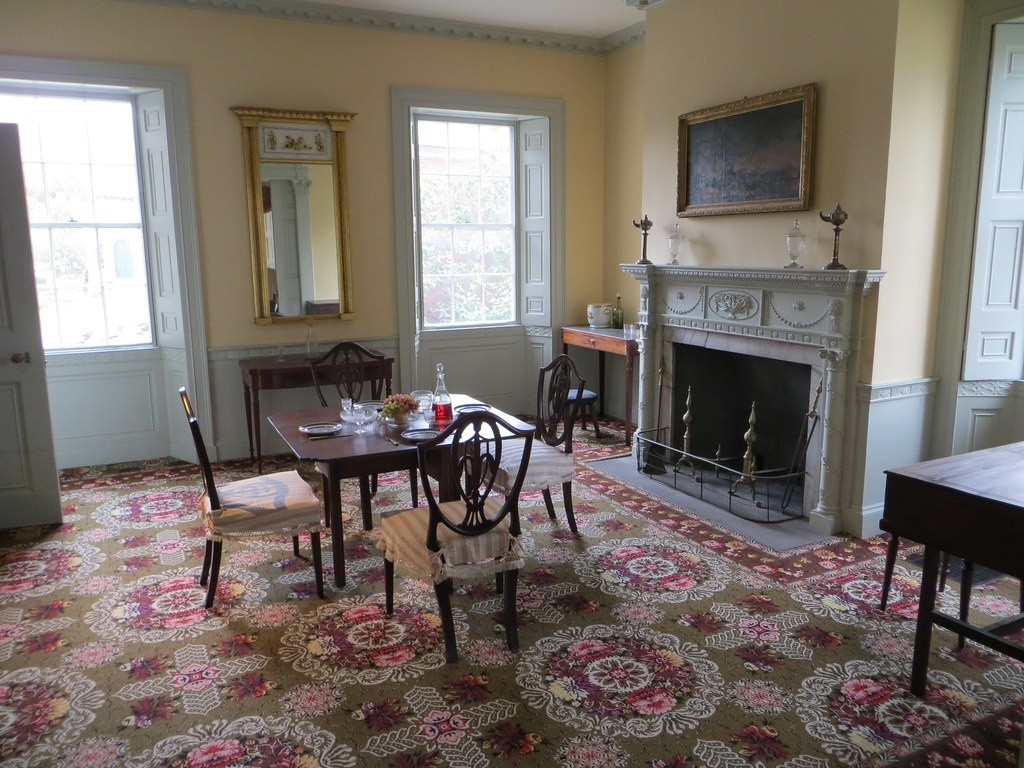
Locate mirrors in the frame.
[228,106,359,326]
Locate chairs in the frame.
[465,353,585,533]
[381,411,536,665]
[178,386,325,609]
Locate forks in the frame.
[383,433,398,445]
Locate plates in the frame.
[454,404,491,414]
[339,410,378,423]
[354,400,384,410]
[298,422,342,435]
[401,428,443,441]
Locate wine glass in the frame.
[352,410,366,434]
[667,223,685,265]
[784,219,806,269]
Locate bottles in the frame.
[305,323,320,360]
[613,296,623,329]
[432,363,453,425]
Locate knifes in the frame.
[309,434,354,440]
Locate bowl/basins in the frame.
[411,390,433,411]
[587,303,613,328]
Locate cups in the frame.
[341,398,352,411]
[423,409,436,423]
[624,323,638,340]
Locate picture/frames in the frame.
[676,82,816,218]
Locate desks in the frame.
[239,349,395,475]
[308,341,418,530]
[560,326,637,446]
[876,440,1024,701]
[266,393,534,588]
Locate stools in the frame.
[565,389,602,438]
[879,519,1024,648]
